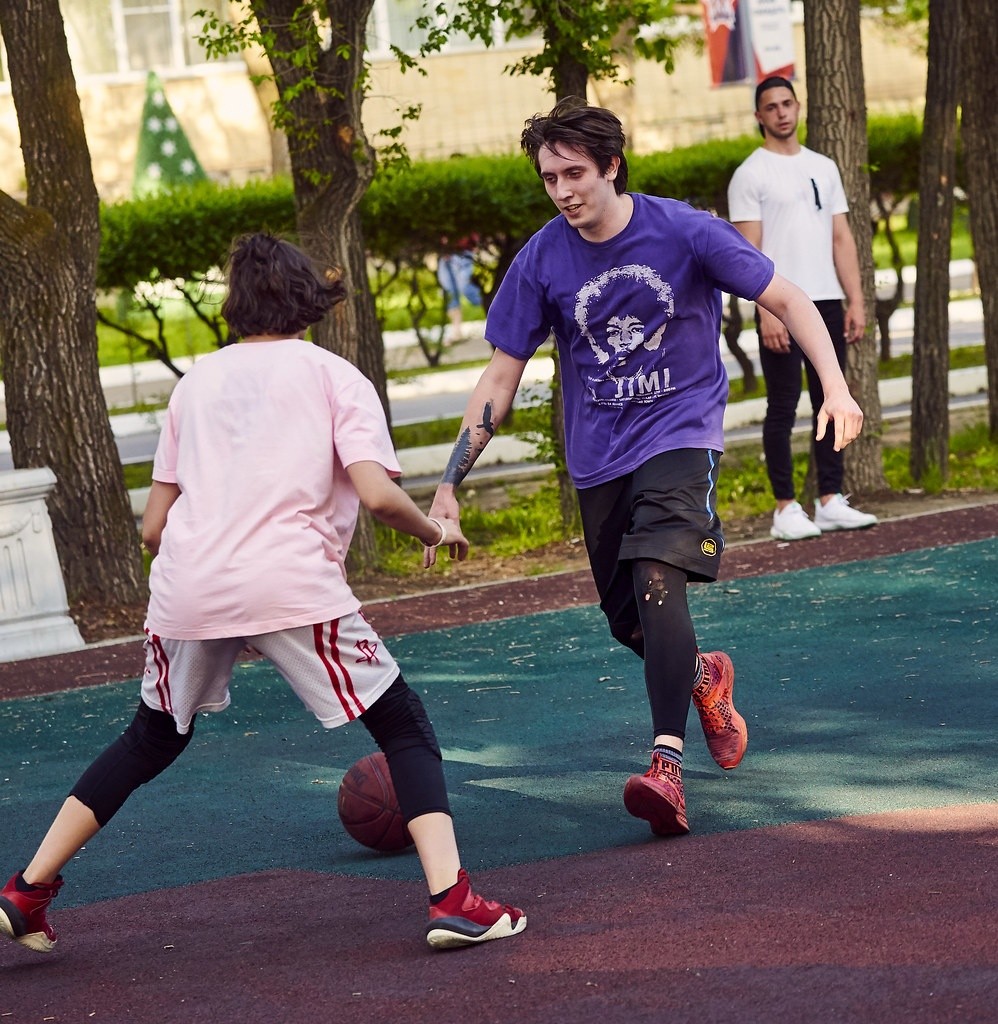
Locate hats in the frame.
[756,76,795,140]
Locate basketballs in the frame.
[336,751,419,851]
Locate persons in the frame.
[0,234,527,951]
[433,227,482,346]
[429,104,864,836]
[727,77,878,540]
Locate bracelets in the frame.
[422,517,446,549]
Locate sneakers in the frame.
[813,492,878,532]
[426,867,528,948]
[693,650,748,770]
[623,752,690,836]
[770,499,822,541]
[0,869,65,952]
